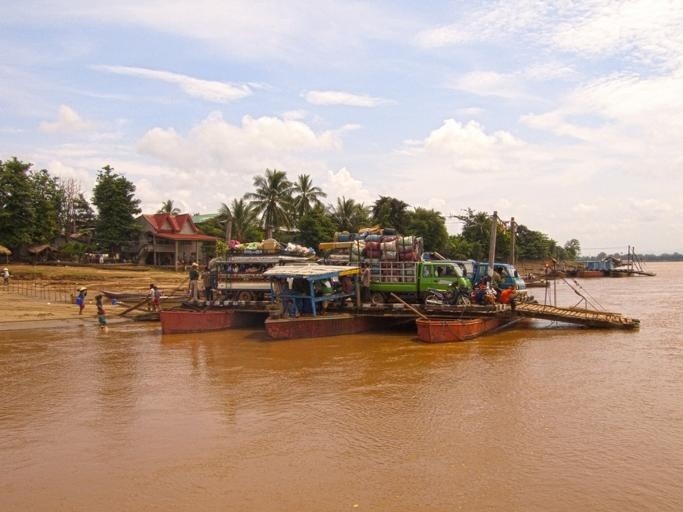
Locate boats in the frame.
[416,315,524,342]
[102,290,187,303]
[526,269,604,287]
[264,314,413,339]
[160,306,259,334]
[613,271,634,277]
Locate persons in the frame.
[153,286,162,312]
[285,277,354,312]
[75,286,87,315]
[200,267,214,301]
[94,293,105,315]
[185,262,200,300]
[0,267,9,286]
[469,268,520,303]
[283,299,298,319]
[147,283,157,312]
[434,267,443,276]
[218,263,273,275]
[79,250,120,264]
[359,261,370,302]
[445,267,453,274]
